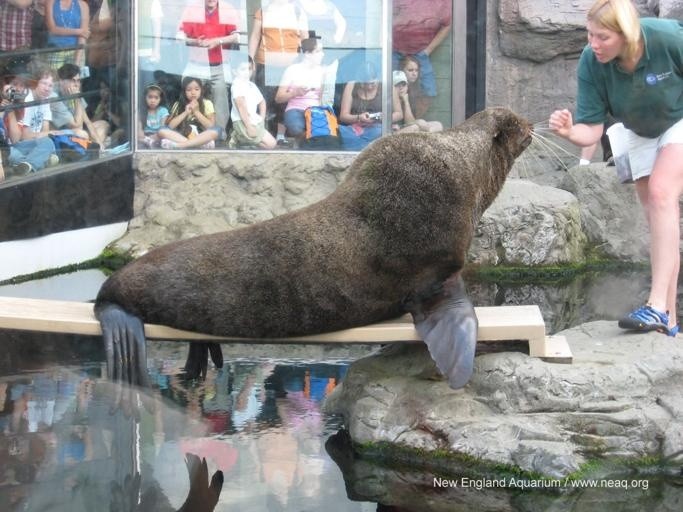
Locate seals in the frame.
[93,105,586,424]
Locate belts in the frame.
[190,61,221,66]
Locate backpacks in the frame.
[48,132,91,161]
[294,105,342,152]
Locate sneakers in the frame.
[200,140,215,149]
[220,129,227,141]
[228,136,236,149]
[145,137,154,148]
[88,143,101,162]
[160,138,173,150]
[12,153,59,176]
[617,306,668,331]
[276,133,285,145]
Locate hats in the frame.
[392,70,407,85]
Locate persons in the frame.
[578,111,618,166]
[548,1,683,336]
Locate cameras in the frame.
[368,111,382,120]
[7,88,25,104]
